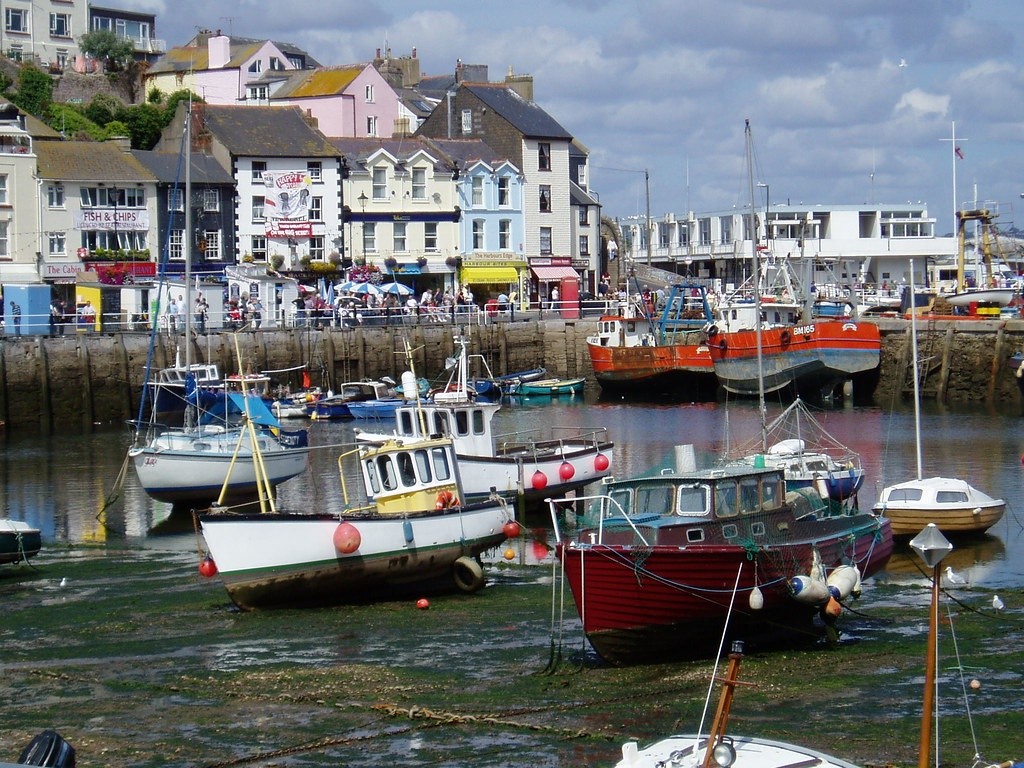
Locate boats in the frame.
[0,518,42,566]
[353,270,614,512]
[873,252,1008,543]
[190,324,515,611]
[543,468,895,670]
[613,524,1024,768]
[223,287,586,418]
[586,121,1024,398]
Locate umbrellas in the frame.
[350,281,382,294]
[334,281,358,292]
[378,281,414,302]
[300,277,334,303]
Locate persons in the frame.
[50,301,95,337]
[224,291,267,328]
[811,282,819,293]
[882,281,904,295]
[292,294,418,327]
[951,277,957,289]
[607,237,618,263]
[993,277,997,287]
[599,272,627,300]
[692,287,716,301]
[10,302,20,337]
[419,286,473,322]
[164,292,209,333]
[551,286,560,308]
[642,285,665,303]
[498,292,516,310]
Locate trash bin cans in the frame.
[488,299,498,317]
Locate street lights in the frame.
[356,191,369,266]
[110,186,121,264]
[756,182,769,293]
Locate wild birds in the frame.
[899,56,909,68]
[993,595,1006,614]
[944,567,969,587]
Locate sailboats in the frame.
[586,118,866,528]
[124,52,309,504]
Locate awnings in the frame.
[461,267,580,284]
